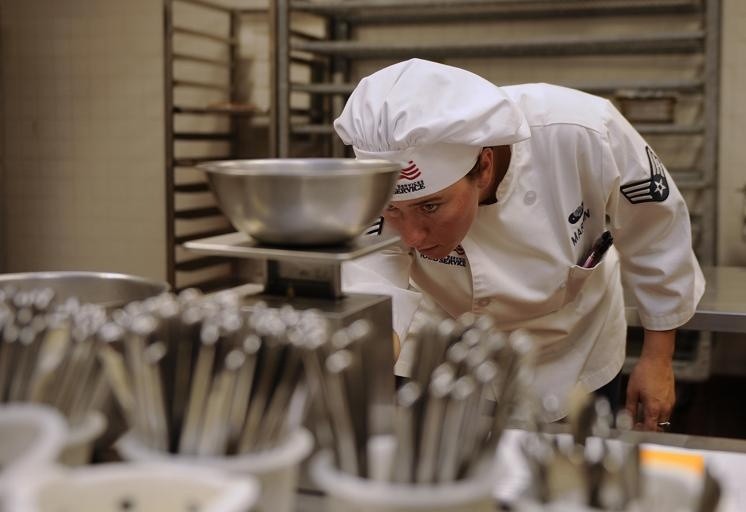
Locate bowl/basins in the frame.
[0,268,170,316]
[196,156,402,247]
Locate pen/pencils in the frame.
[582,231,613,271]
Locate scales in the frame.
[182,157,405,420]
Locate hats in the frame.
[332,56,532,203]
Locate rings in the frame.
[656,420,671,429]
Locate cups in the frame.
[107,421,317,509]
[25,467,256,512]
[307,430,517,510]
[2,402,55,511]
[500,465,723,512]
[52,411,105,463]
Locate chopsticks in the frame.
[1,282,533,483]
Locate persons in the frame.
[340,56,705,434]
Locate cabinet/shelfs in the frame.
[279,4,721,271]
